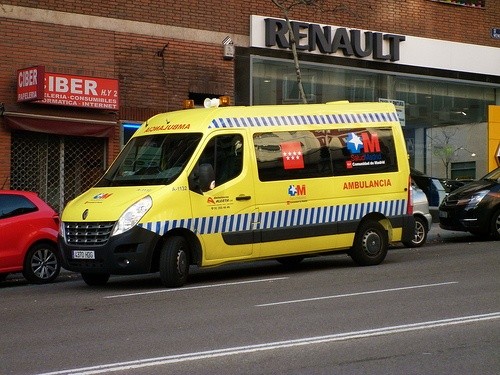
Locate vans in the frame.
[56,93,414,287]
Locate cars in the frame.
[0,188,63,284]
[411,180,433,247]
[437,166,500,241]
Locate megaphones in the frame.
[204,98,219,108]
